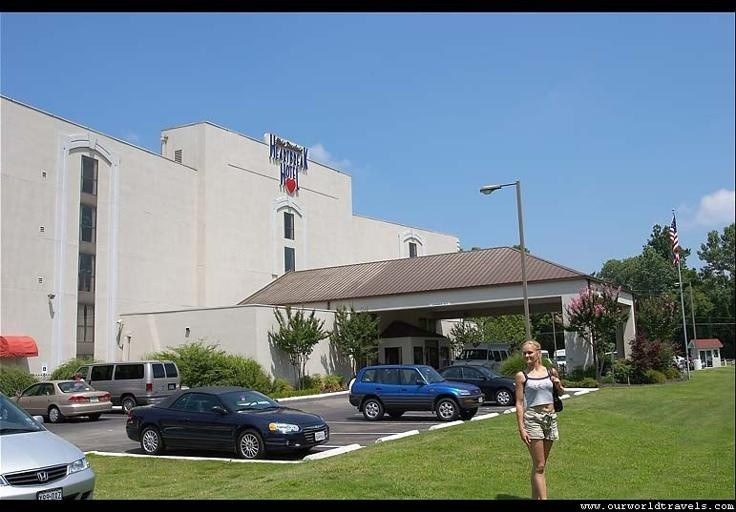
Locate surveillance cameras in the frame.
[116,320,124,326]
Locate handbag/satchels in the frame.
[553,383,563,411]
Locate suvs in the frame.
[349,364,485,422]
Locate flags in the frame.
[668,215,679,267]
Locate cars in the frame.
[9,379,112,420]
[0,392,95,502]
[126,386,329,459]
[437,365,517,406]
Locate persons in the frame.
[516,341,564,500]
[72,373,86,389]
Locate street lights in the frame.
[675,281,696,339]
[479,180,531,340]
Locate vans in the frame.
[540,349,567,367]
[452,341,511,369]
[73,359,179,413]
